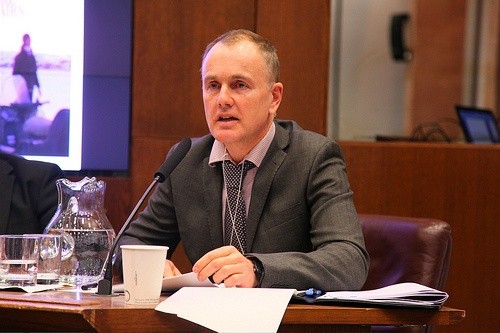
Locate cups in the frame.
[23,234,63,284]
[120,244,170,304]
[0,235,39,287]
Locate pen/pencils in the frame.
[81,282,98,290]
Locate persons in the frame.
[0,150,67,268]
[112,29,370,292]
[12,34,43,104]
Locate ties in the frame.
[222,159,255,254]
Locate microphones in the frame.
[96,137,193,294]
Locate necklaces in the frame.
[222,147,244,257]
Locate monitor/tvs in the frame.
[454,104,500,144]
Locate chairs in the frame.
[358,213,453,294]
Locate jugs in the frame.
[40,176,117,288]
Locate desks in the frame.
[336,141,500,333]
[0,286,465,333]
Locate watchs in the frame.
[250,258,266,283]
